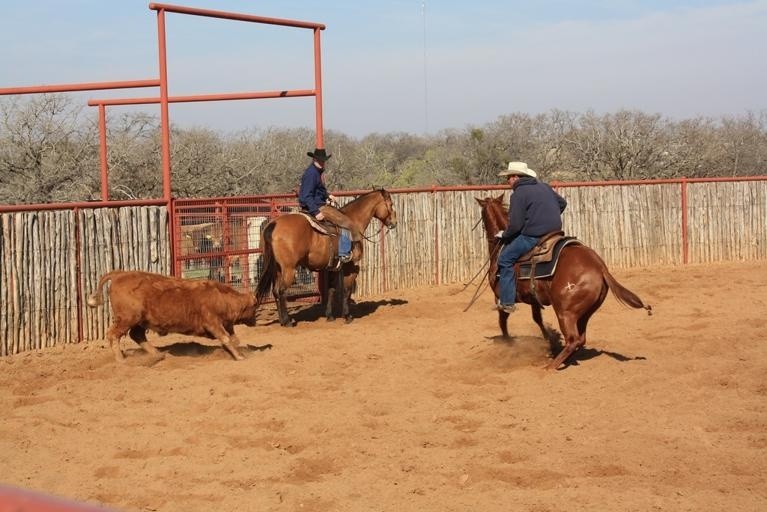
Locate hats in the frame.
[307,148,332,162]
[496,161,538,178]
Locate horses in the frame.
[253,185,398,327]
[474,191,644,372]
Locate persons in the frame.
[492,161,567,314]
[298,149,352,264]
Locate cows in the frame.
[86,269,257,361]
[181,217,312,284]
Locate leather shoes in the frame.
[491,302,515,312]
